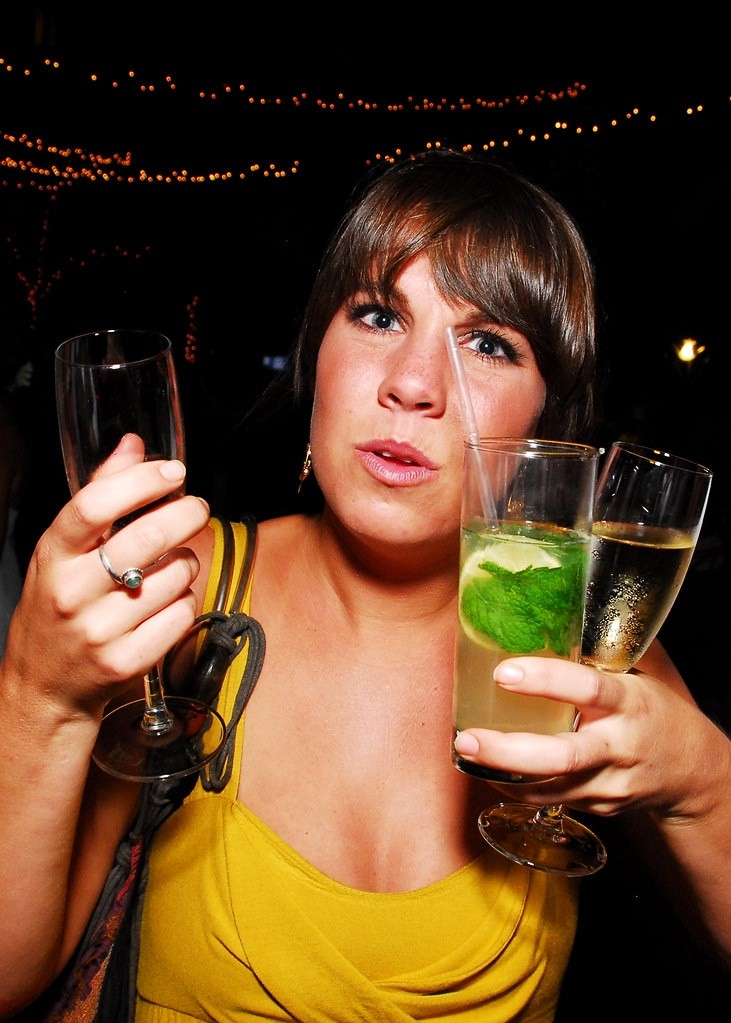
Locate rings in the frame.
[98,545,143,588]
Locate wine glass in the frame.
[477,442,716,879]
[54,328,226,783]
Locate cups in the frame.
[449,438,599,785]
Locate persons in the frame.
[0,148,731,1023]
